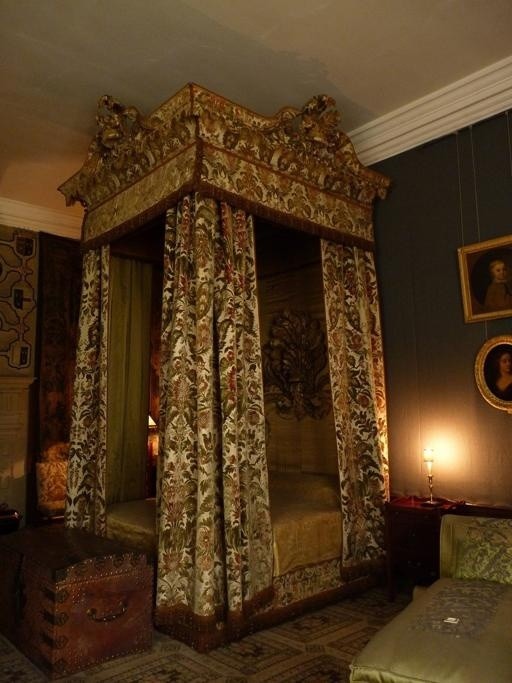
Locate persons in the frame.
[493,351,512,402]
[484,260,512,312]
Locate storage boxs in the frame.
[0,524,154,680]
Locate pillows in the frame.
[267,470,341,507]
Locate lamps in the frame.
[420,475,443,507]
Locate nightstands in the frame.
[383,496,464,602]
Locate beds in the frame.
[57,82,391,653]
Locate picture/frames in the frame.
[474,335,512,415]
[456,234,512,325]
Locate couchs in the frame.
[348,515,512,683]
